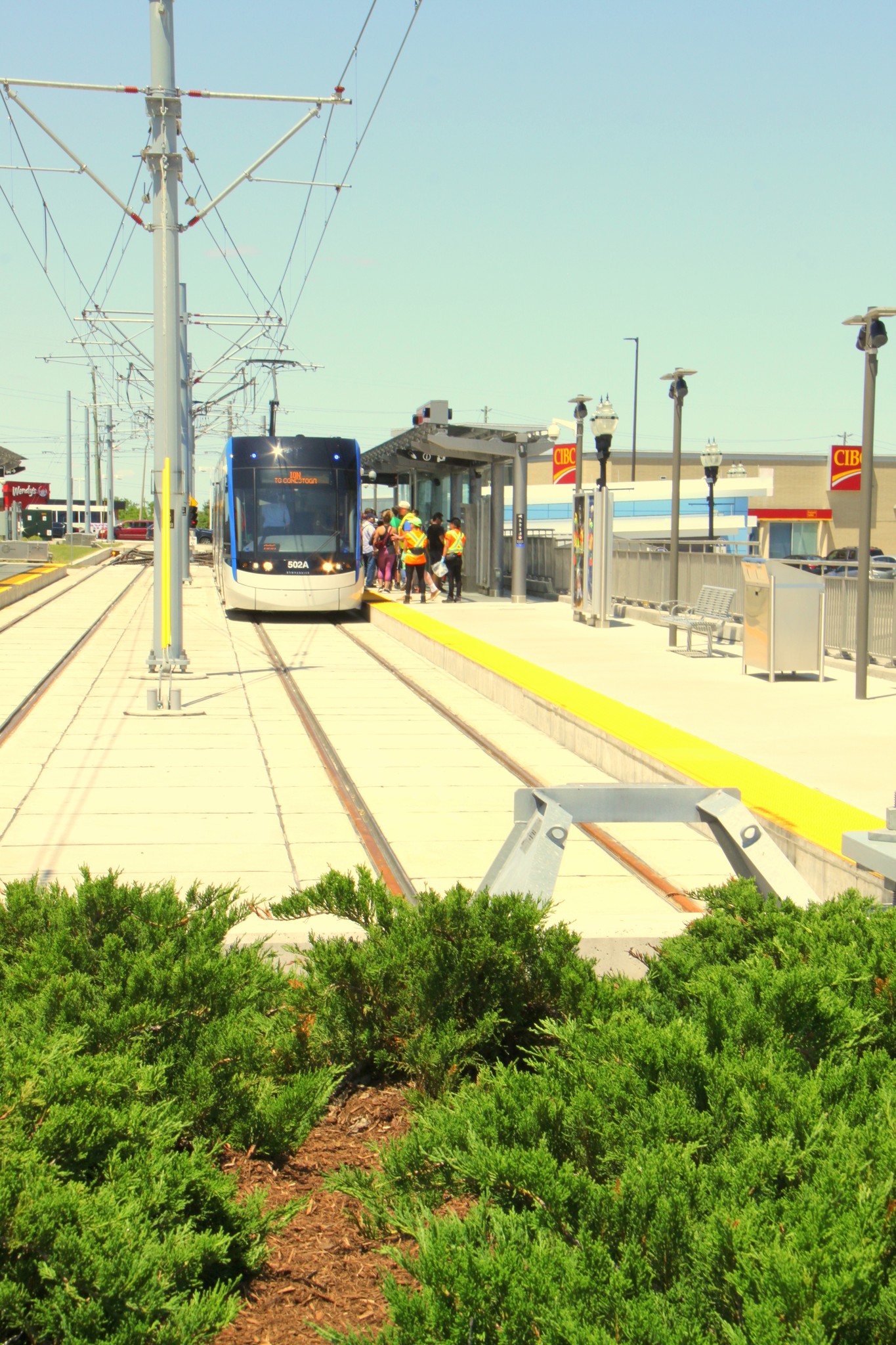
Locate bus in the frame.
[210,434,365,615]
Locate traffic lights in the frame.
[187,506,198,529]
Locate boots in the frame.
[421,595,426,603]
[403,596,410,604]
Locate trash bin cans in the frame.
[742,557,826,671]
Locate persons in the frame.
[372,510,401,594]
[361,508,425,593]
[361,513,377,588]
[262,495,290,536]
[426,512,447,593]
[439,517,466,603]
[390,500,442,602]
[402,518,430,604]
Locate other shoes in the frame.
[394,582,401,589]
[366,585,377,588]
[401,583,406,591]
[378,586,390,593]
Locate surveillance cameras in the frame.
[368,471,377,482]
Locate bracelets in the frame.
[397,538,399,540]
[373,550,376,552]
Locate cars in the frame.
[52,522,80,538]
[780,555,825,576]
[822,555,896,580]
[146,521,213,545]
[97,520,154,541]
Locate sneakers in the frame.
[454,596,461,603]
[442,596,454,603]
[396,595,412,600]
[428,589,441,601]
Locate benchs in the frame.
[660,584,735,658]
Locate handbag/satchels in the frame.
[408,547,424,555]
[431,561,448,577]
[374,527,392,550]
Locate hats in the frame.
[365,513,378,520]
[408,518,422,527]
[446,517,461,528]
[398,501,410,509]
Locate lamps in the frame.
[518,442,527,457]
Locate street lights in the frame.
[698,436,723,553]
[567,394,592,608]
[841,306,896,703]
[622,336,639,482]
[659,367,699,647]
[590,394,620,492]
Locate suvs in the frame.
[825,546,885,572]
